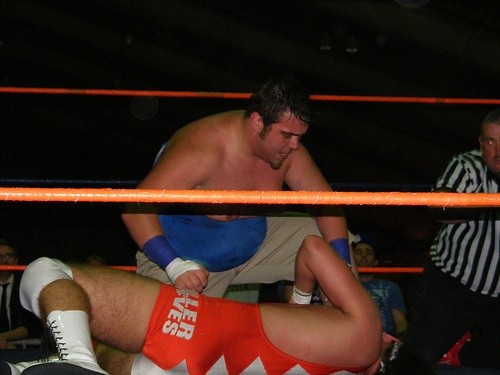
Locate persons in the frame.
[399,107,500,368]
[0,234,436,375]
[354,240,407,332]
[0,241,43,349]
[120,74,359,305]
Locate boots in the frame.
[0,353,59,375]
[20,310,110,375]
